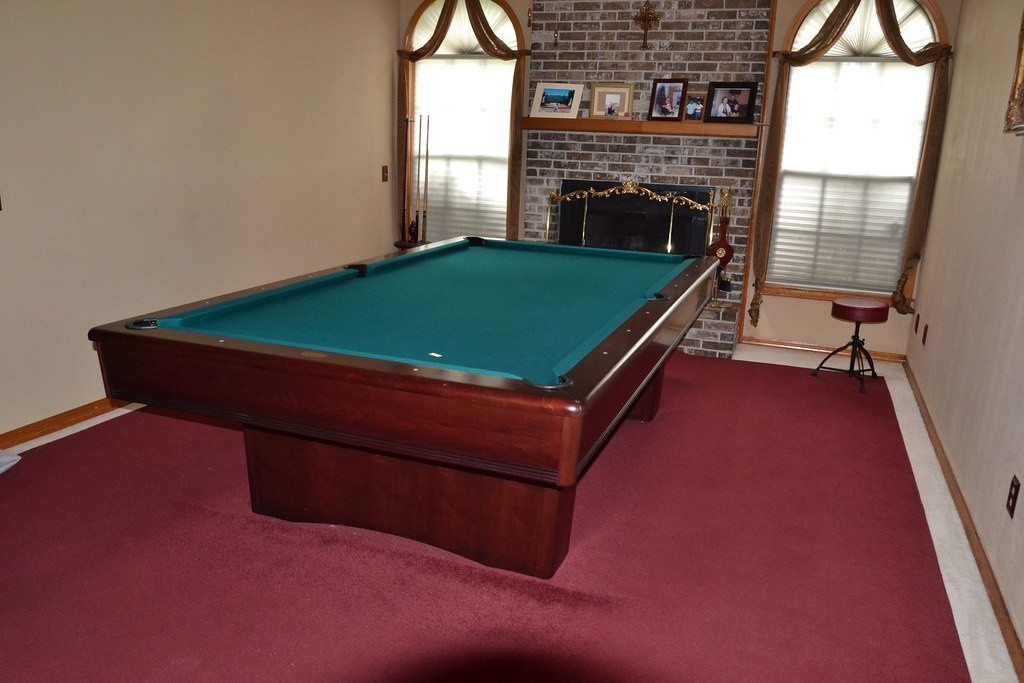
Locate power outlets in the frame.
[1005,474,1020,520]
[922,323,928,346]
[915,313,920,333]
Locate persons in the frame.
[553,102,559,112]
[686,99,703,120]
[717,96,740,117]
[607,105,615,116]
[660,96,675,117]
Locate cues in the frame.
[414,115,422,245]
[401,113,408,243]
[422,114,430,243]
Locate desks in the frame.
[88,236,721,581]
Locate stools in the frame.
[810,298,890,396]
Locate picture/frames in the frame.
[647,78,689,121]
[683,92,707,123]
[529,82,585,119]
[589,83,635,121]
[702,81,759,123]
